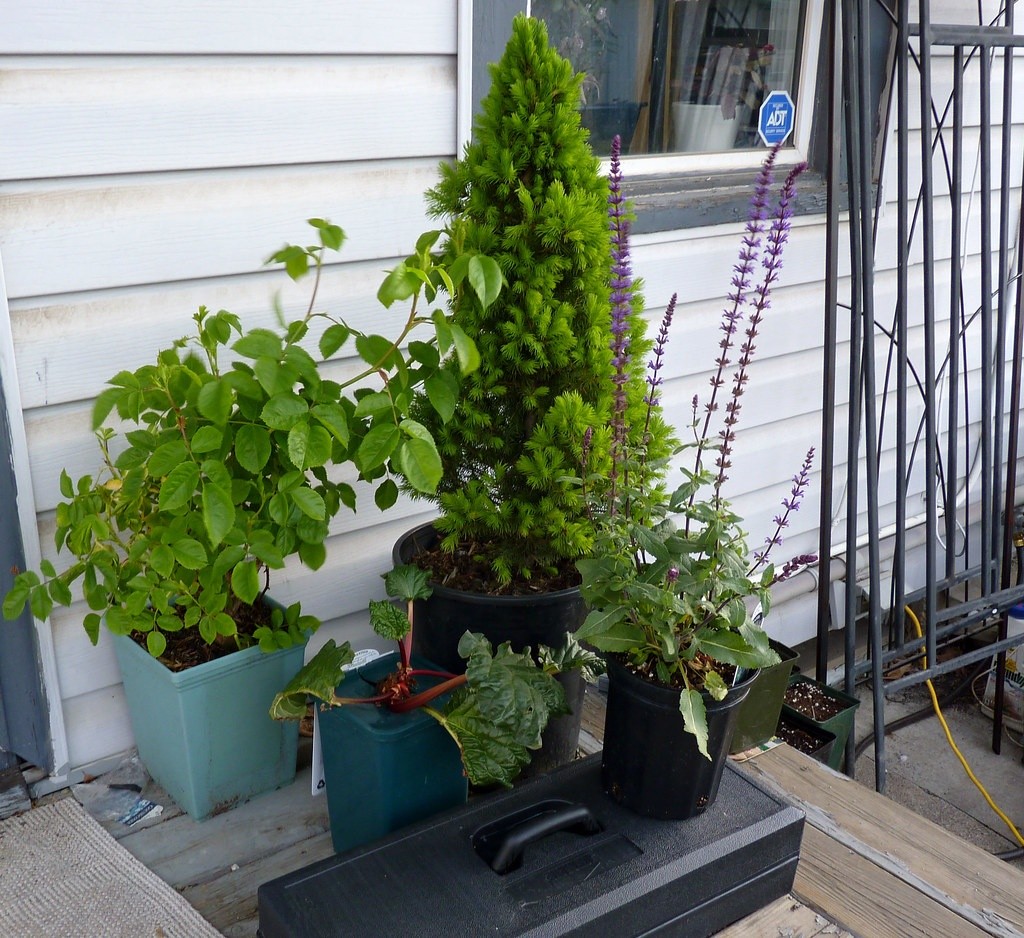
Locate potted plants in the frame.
[0,214,509,817]
[670,42,775,150]
[385,14,683,785]
[277,552,565,847]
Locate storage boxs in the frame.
[251,725,808,938]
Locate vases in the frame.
[728,635,803,748]
[785,674,861,775]
[601,626,761,821]
[778,712,838,768]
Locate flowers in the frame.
[572,127,816,772]
